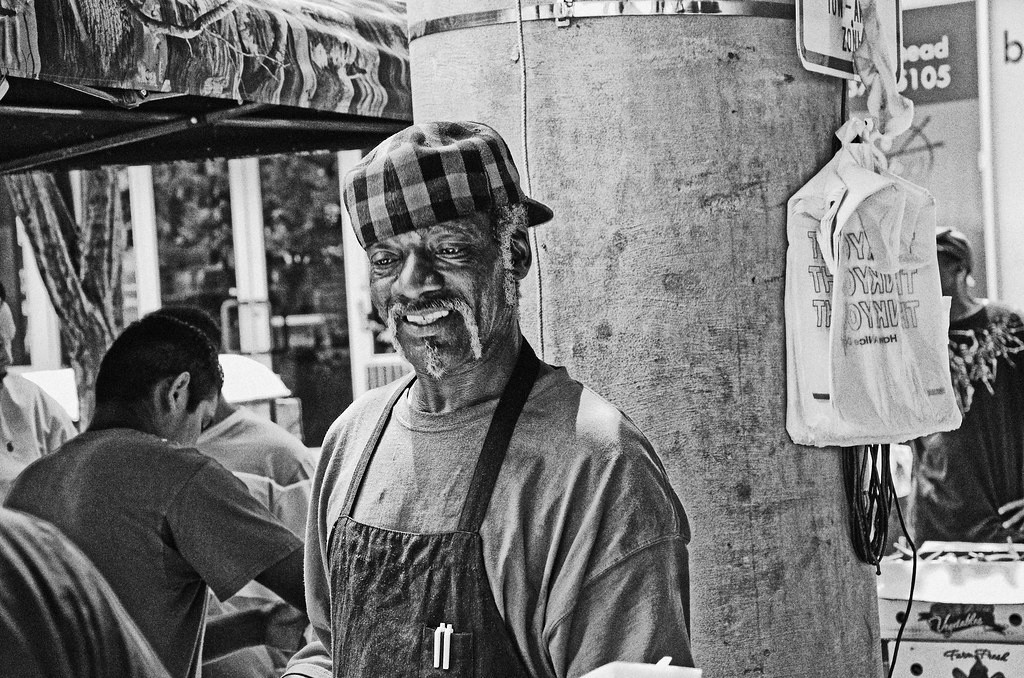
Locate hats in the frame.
[342,121,554,252]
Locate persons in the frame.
[0,121,690,678]
[906,225,1024,549]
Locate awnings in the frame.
[0,0,413,121]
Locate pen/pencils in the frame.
[433,623,454,669]
